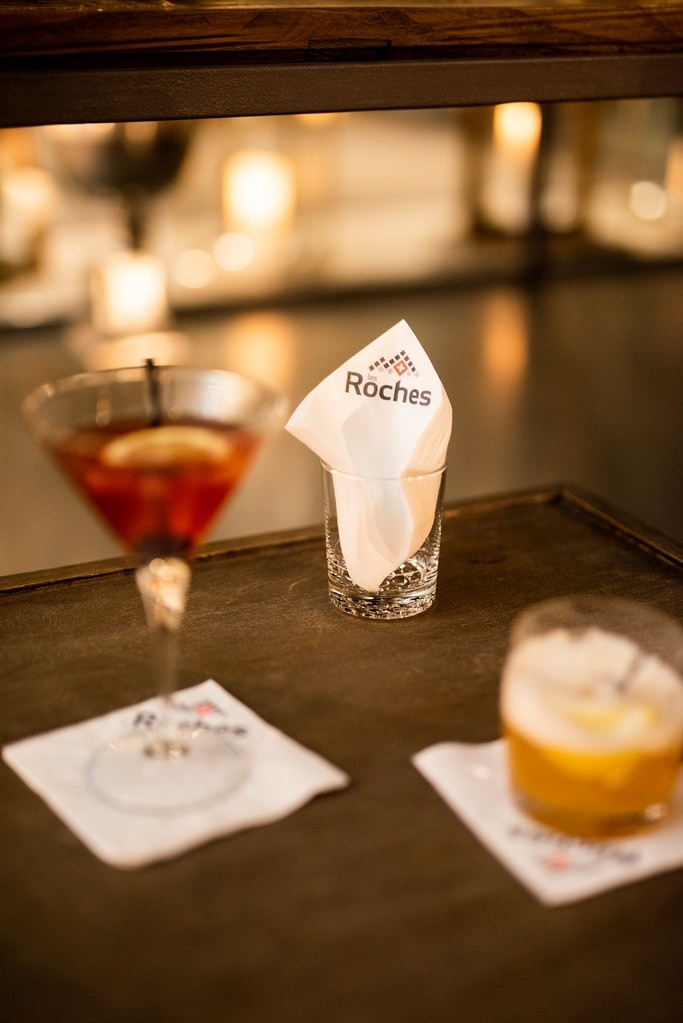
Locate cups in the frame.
[502,592,682,841]
[317,456,448,621]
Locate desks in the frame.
[0,479,683,1023]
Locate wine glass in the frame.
[21,354,287,814]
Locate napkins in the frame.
[285,318,452,591]
[410,731,683,909]
[1,679,350,872]
